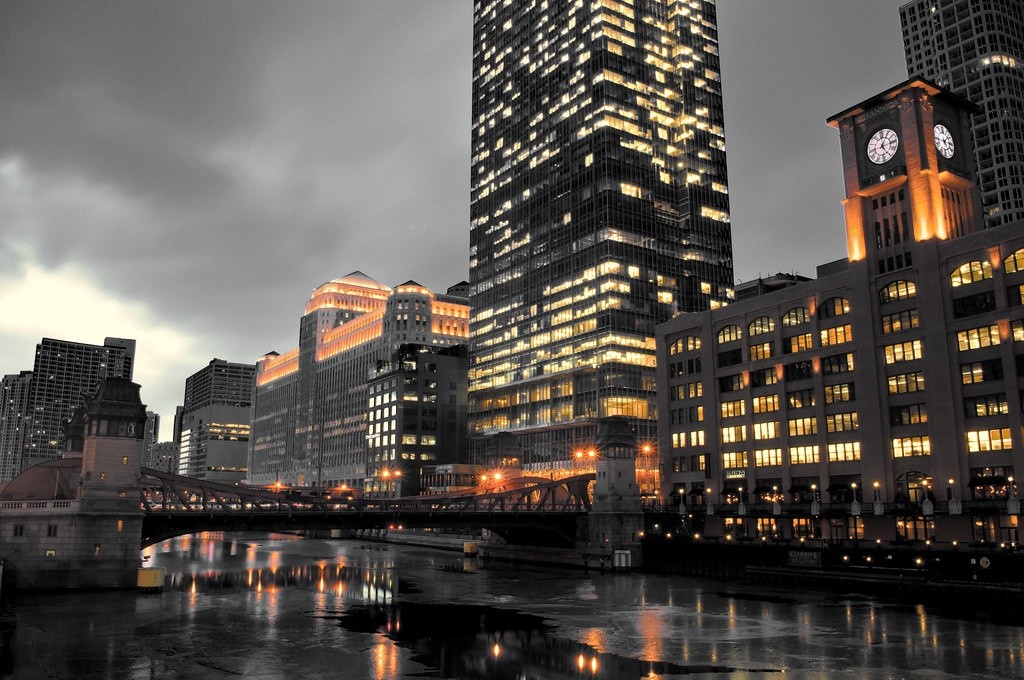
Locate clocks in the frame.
[934,124,955,159]
[867,129,899,165]
